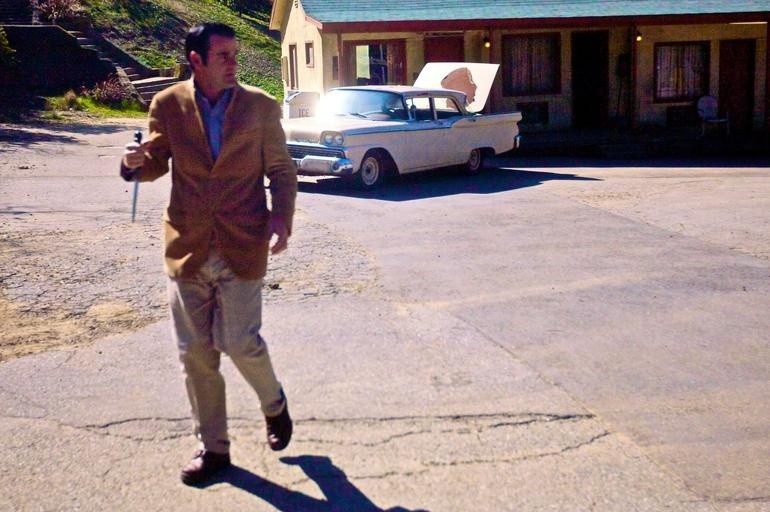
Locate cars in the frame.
[279,61,523,190]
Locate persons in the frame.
[119,22,299,485]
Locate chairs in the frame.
[696,95,730,137]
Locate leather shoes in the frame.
[182,450,231,483]
[265,408,293,451]
